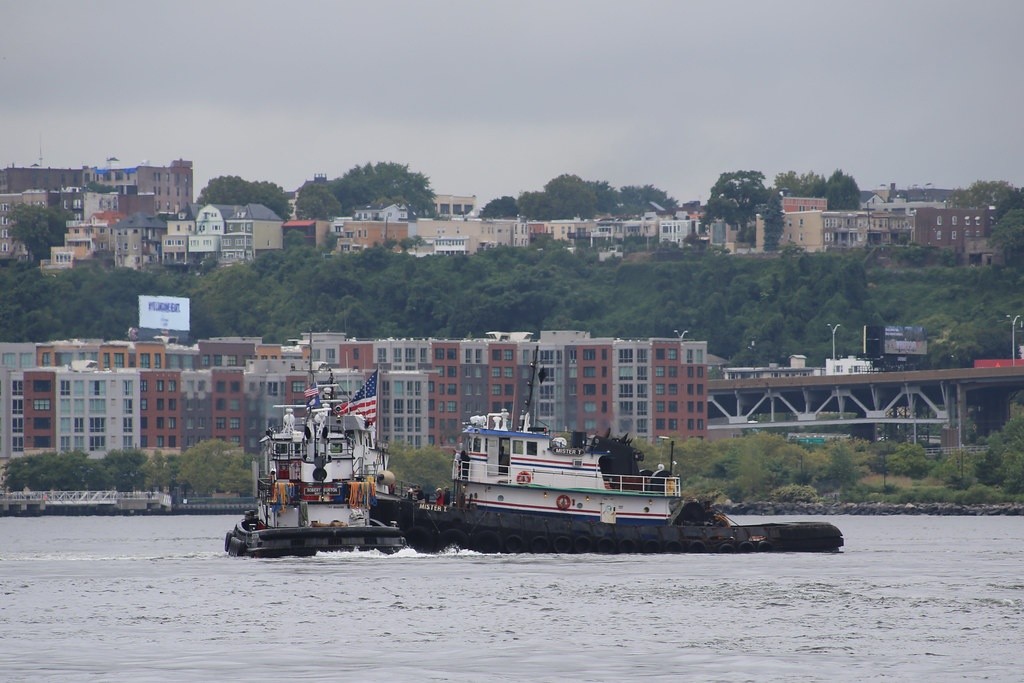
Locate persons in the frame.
[388,450,471,506]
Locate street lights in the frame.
[674,329,689,338]
[827,323,841,375]
[1006,314,1021,367]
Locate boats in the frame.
[372,346,845,555]
[226,331,405,558]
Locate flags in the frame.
[331,369,379,433]
[304,382,322,409]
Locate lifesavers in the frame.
[517,471,532,485]
[556,495,571,509]
